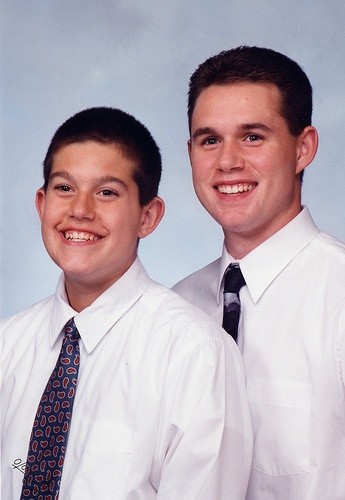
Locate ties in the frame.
[221,265,245,344]
[22,317,80,499]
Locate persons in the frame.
[169,44,345,500]
[0,107,253,500]
